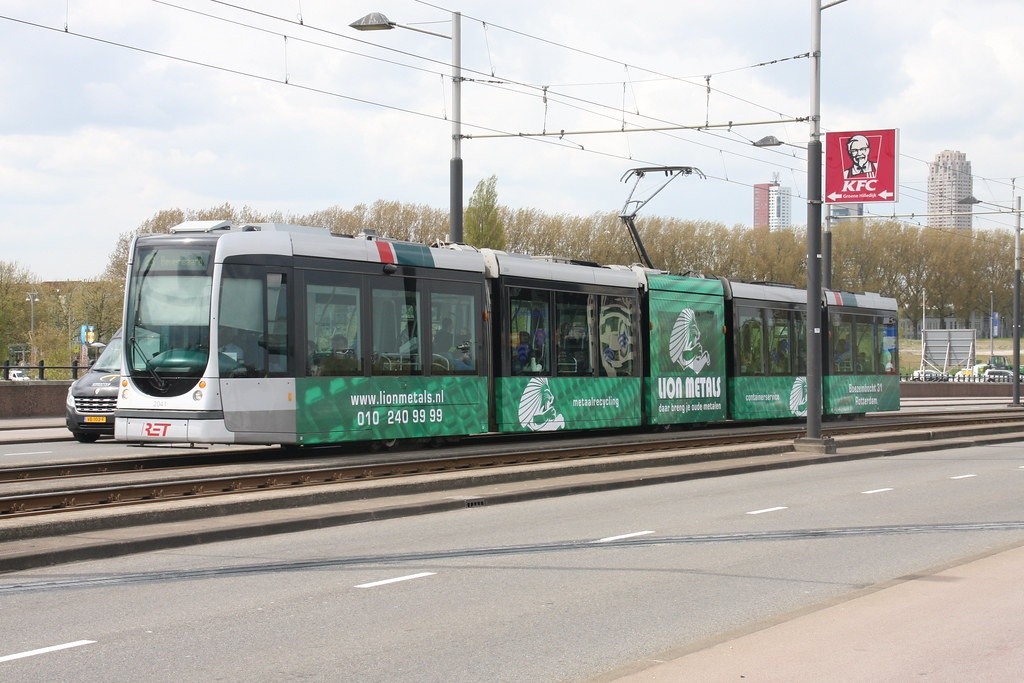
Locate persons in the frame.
[557,323,575,363]
[218,327,245,364]
[516,331,532,367]
[321,334,357,376]
[431,317,455,354]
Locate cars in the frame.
[910,356,1024,382]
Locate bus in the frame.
[114,221,901,452]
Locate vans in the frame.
[65,326,122,443]
[8,371,30,381]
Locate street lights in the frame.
[26,287,40,367]
[752,136,832,292]
[957,196,1024,407]
[348,12,464,245]
